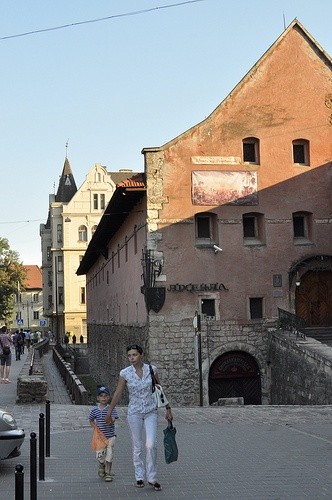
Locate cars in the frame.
[0,410,24,460]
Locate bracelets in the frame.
[166,406,171,410]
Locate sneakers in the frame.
[136,480,144,487]
[97,462,112,481]
[149,482,162,491]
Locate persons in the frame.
[63,331,72,344]
[0,326,14,384]
[80,335,84,344]
[72,334,77,345]
[12,326,42,360]
[89,387,119,482]
[105,344,173,492]
[48,329,55,342]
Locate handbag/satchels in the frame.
[92,425,109,451]
[0,338,10,355]
[162,420,178,464]
[149,365,169,408]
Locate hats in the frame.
[96,386,111,396]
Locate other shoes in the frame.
[1,378,11,384]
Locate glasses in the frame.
[126,345,138,349]
